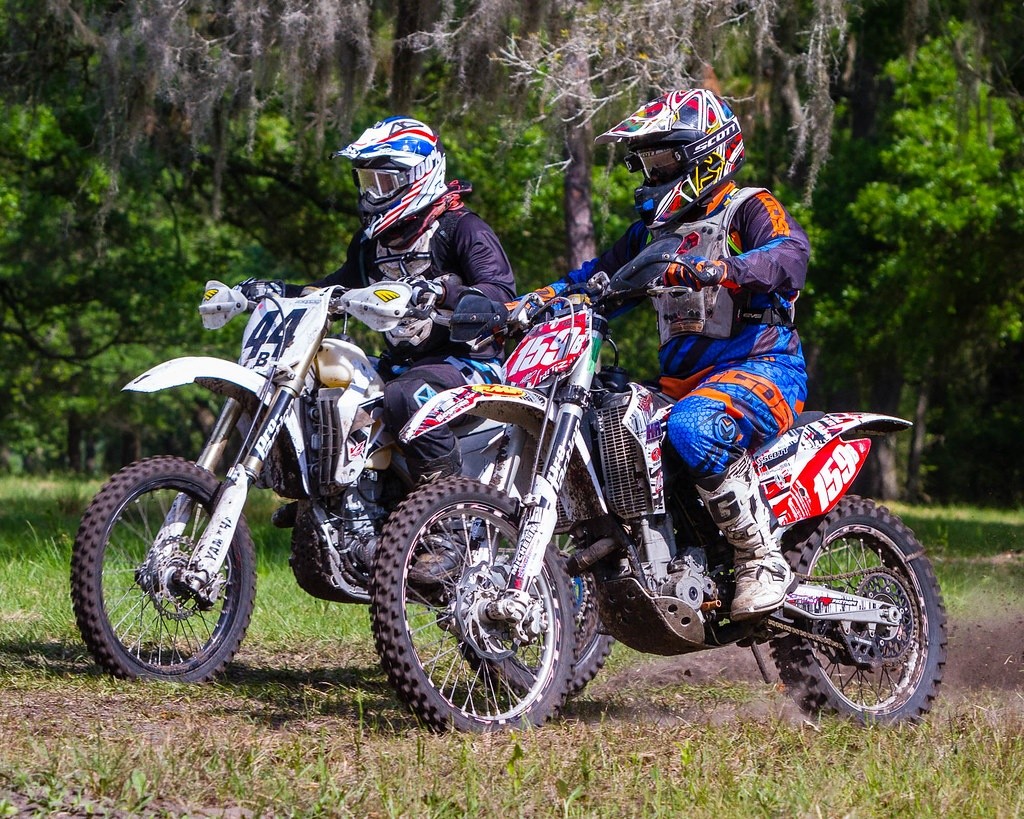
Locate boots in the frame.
[407,436,480,584]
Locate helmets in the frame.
[593,89,748,229]
[330,116,449,240]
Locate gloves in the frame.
[503,287,556,317]
[662,254,734,292]
[232,277,285,304]
[396,276,444,307]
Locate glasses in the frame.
[637,148,680,182]
[356,168,410,201]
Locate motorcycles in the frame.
[368,233,949,735]
[70,280,614,685]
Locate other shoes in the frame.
[695,449,799,621]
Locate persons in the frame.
[241,111,518,582]
[501,88,812,622]
